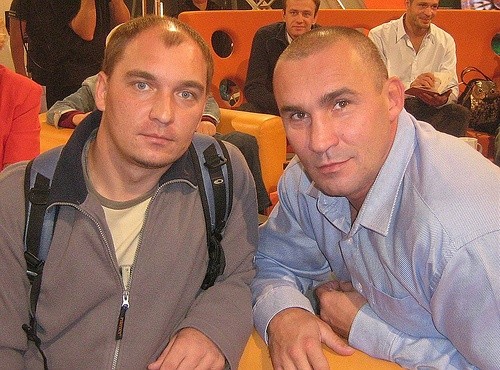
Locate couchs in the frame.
[38,105,287,199]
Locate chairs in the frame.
[238,326,404,370]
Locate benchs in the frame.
[179,8,499,158]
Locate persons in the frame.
[251,25,500,370]
[9,0,130,110]
[0,14,259,370]
[47,22,272,215]
[367,0,471,137]
[175,0,219,16]
[0,63,44,173]
[243,0,323,116]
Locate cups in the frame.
[459,137,482,154]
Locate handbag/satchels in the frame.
[458,64,500,136]
